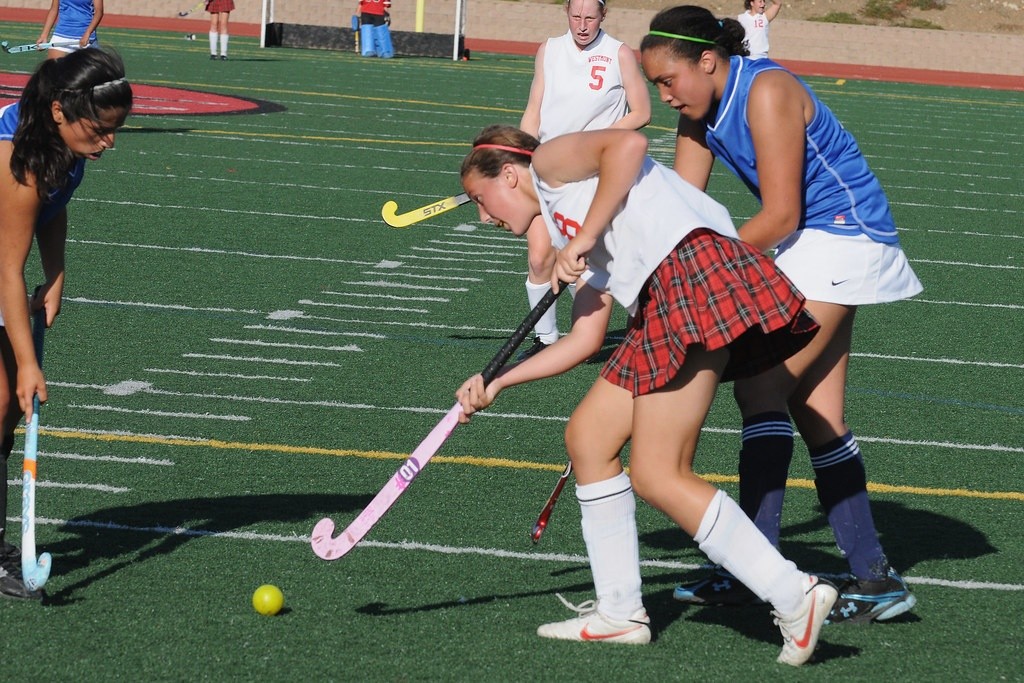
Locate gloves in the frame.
[383,12,392,26]
[351,14,361,32]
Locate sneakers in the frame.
[671,560,754,605]
[514,336,546,364]
[816,563,918,628]
[0,562,43,602]
[0,527,22,564]
[536,591,653,645]
[774,573,839,668]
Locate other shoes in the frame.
[209,54,217,61]
[382,51,395,60]
[220,54,228,60]
[364,51,377,59]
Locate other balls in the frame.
[254,584,284,616]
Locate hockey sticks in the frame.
[355,31,360,54]
[381,191,472,228]
[308,255,590,561]
[19,282,54,593]
[530,461,572,544]
[1,38,95,54]
[179,1,206,16]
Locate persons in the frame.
[640,5,923,620]
[36,0,104,57]
[455,126,839,668]
[0,48,134,601]
[204,0,236,61]
[737,0,782,60]
[351,0,394,58]
[516,0,651,358]
